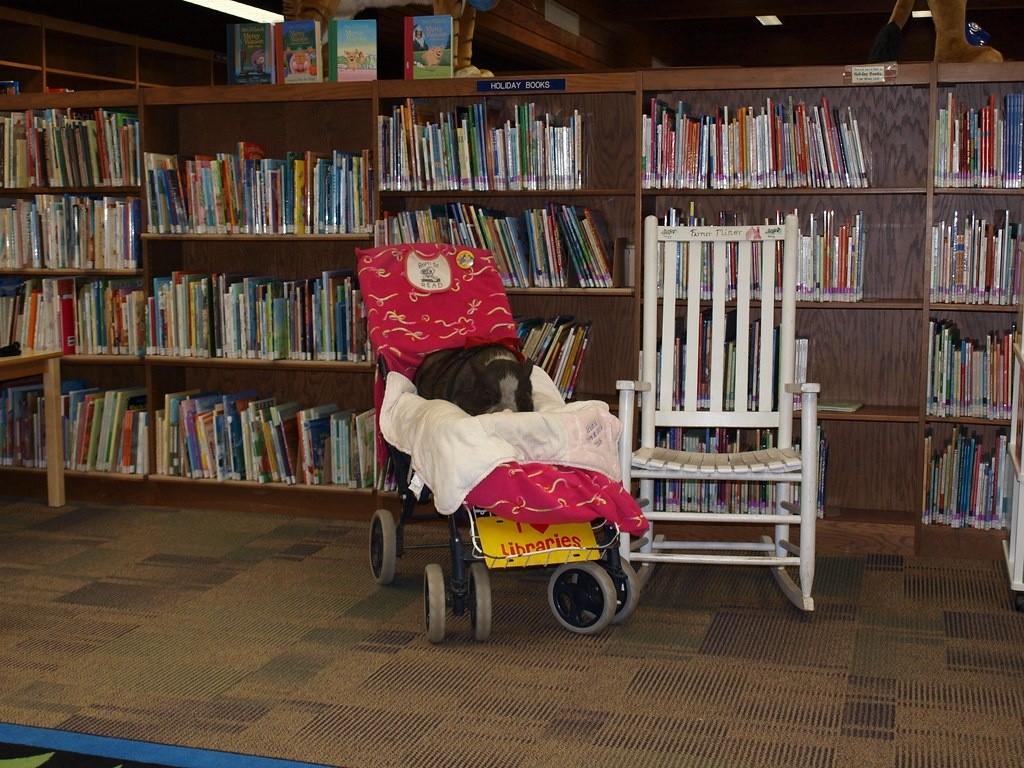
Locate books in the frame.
[0,14,1023,532]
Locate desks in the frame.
[0,348,64,507]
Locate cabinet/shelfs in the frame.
[640,63,934,558]
[142,79,376,522]
[1001,239,1024,613]
[0,87,151,505]
[374,67,639,524]
[917,62,1024,558]
[0,6,228,93]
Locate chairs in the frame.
[615,214,823,612]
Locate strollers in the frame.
[356,241,640,640]
